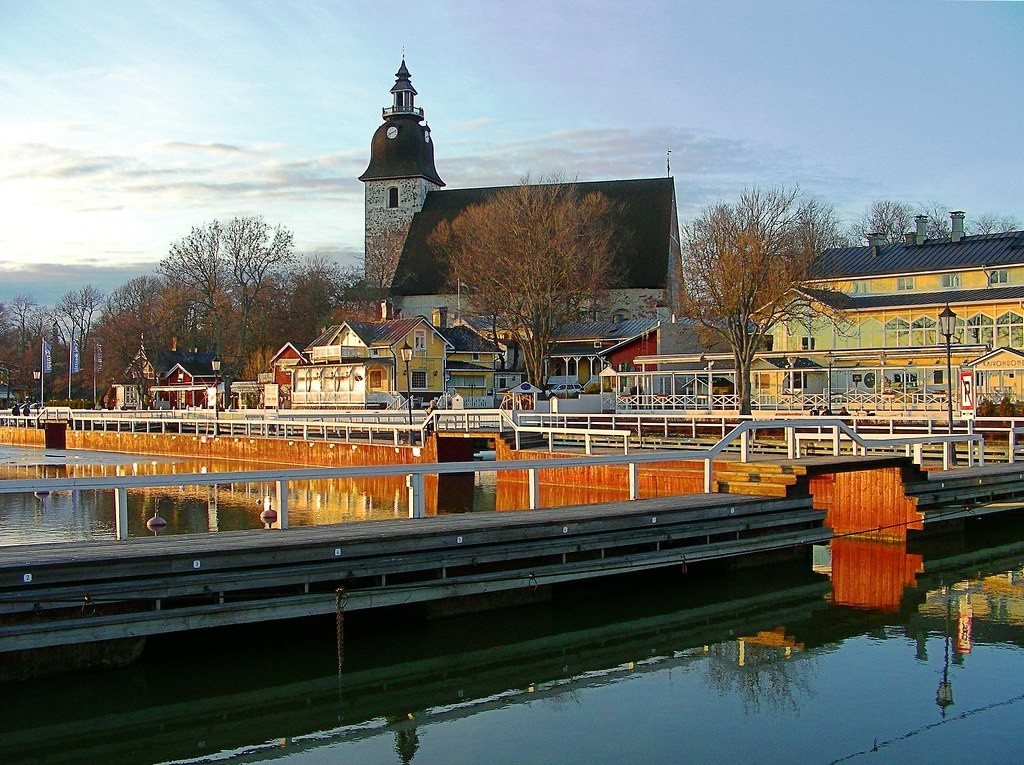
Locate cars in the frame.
[544,384,583,400]
[12,402,47,409]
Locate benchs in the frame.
[560,417,1003,439]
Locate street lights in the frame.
[936,301,958,466]
[33,367,41,429]
[824,351,836,411]
[211,356,221,435]
[400,340,418,445]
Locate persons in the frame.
[821,407,851,424]
[13,404,30,427]
[425,401,439,436]
